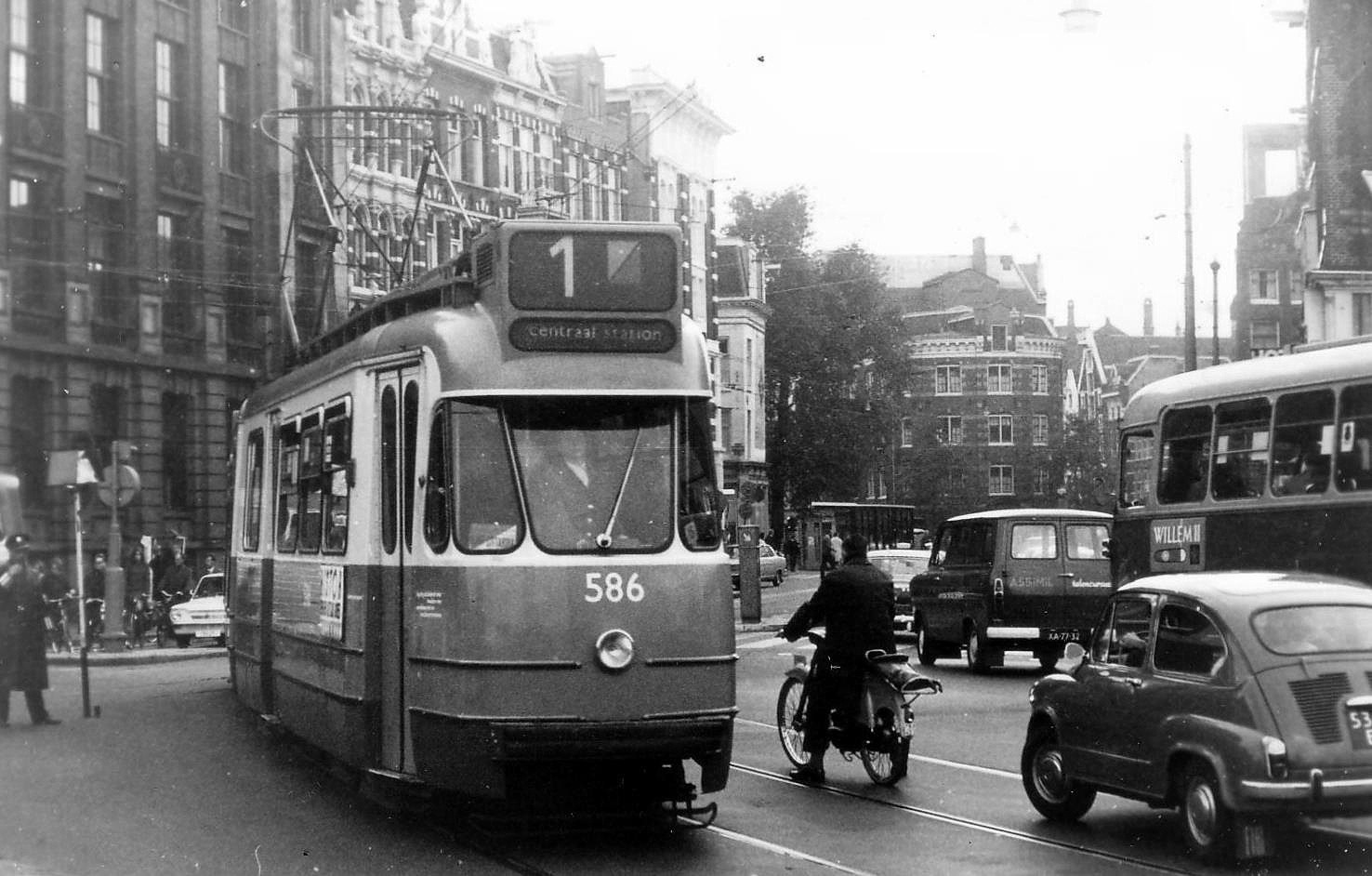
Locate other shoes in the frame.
[0,720,10,727]
[789,766,825,783]
[125,642,133,649]
[147,637,157,643]
[37,716,61,724]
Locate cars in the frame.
[727,542,789,587]
[1019,571,1372,862]
[169,574,232,645]
[859,546,948,635]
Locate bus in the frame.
[1107,346,1372,592]
[169,140,735,829]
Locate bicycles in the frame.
[43,590,80,649]
[123,589,185,649]
[86,596,106,649]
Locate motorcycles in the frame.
[775,630,923,786]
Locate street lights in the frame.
[1210,260,1221,368]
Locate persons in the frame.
[0,533,64,728]
[0,529,223,648]
[757,528,844,574]
[773,535,896,785]
[537,418,643,557]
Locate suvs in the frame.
[909,509,1111,673]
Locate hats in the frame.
[5,534,30,551]
